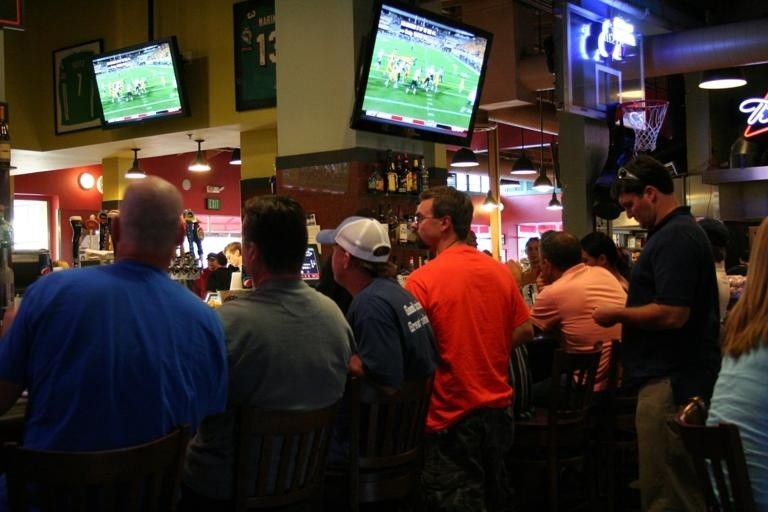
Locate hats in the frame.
[316,215,392,263]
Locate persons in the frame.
[316,214,440,471]
[402,184,535,512]
[726,249,749,277]
[0,174,229,455]
[504,229,643,409]
[697,218,731,325]
[179,193,358,512]
[704,215,768,512]
[376,47,477,108]
[192,241,242,301]
[101,76,166,103]
[590,156,722,512]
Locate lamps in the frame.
[481,192,498,209]
[534,92,554,191]
[187,140,210,172]
[510,128,538,175]
[125,148,145,179]
[546,170,564,211]
[448,148,480,168]
[229,148,242,166]
[696,68,750,90]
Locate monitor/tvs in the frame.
[89,36,192,130]
[350,0,494,146]
[298,244,321,284]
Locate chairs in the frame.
[1,339,767,512]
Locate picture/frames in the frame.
[233,1,277,112]
[53,39,103,134]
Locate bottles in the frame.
[37,250,53,279]
[0,119,12,171]
[0,213,16,247]
[365,150,429,275]
[0,248,15,308]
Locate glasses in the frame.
[617,166,641,181]
[407,214,434,223]
